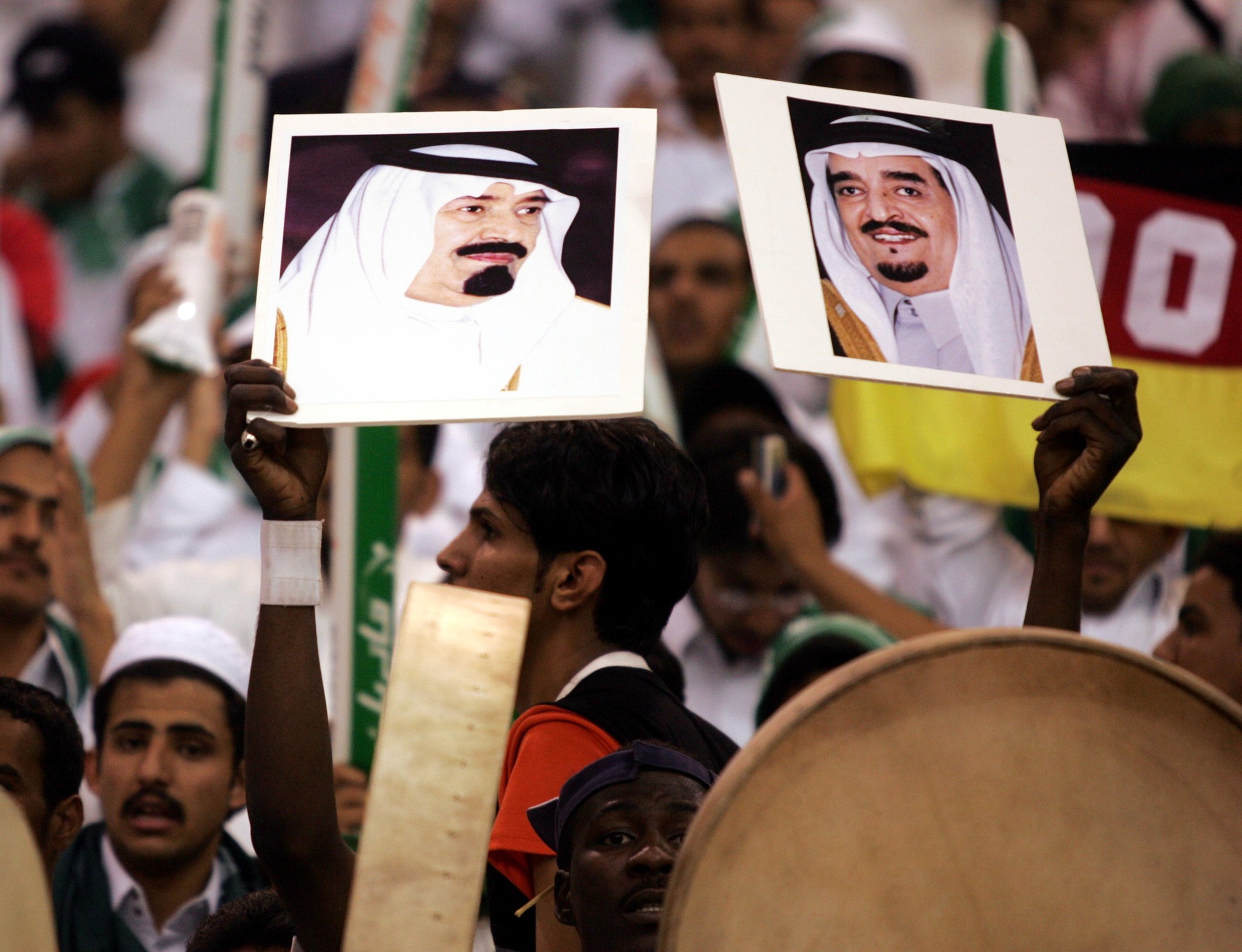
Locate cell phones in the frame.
[751,436,787,536]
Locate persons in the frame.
[0,676,89,887]
[527,741,722,952]
[0,0,1242,835]
[49,616,295,952]
[425,415,750,952]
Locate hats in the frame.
[4,20,134,105]
[530,741,725,865]
[751,609,896,725]
[96,617,251,704]
[797,12,921,98]
